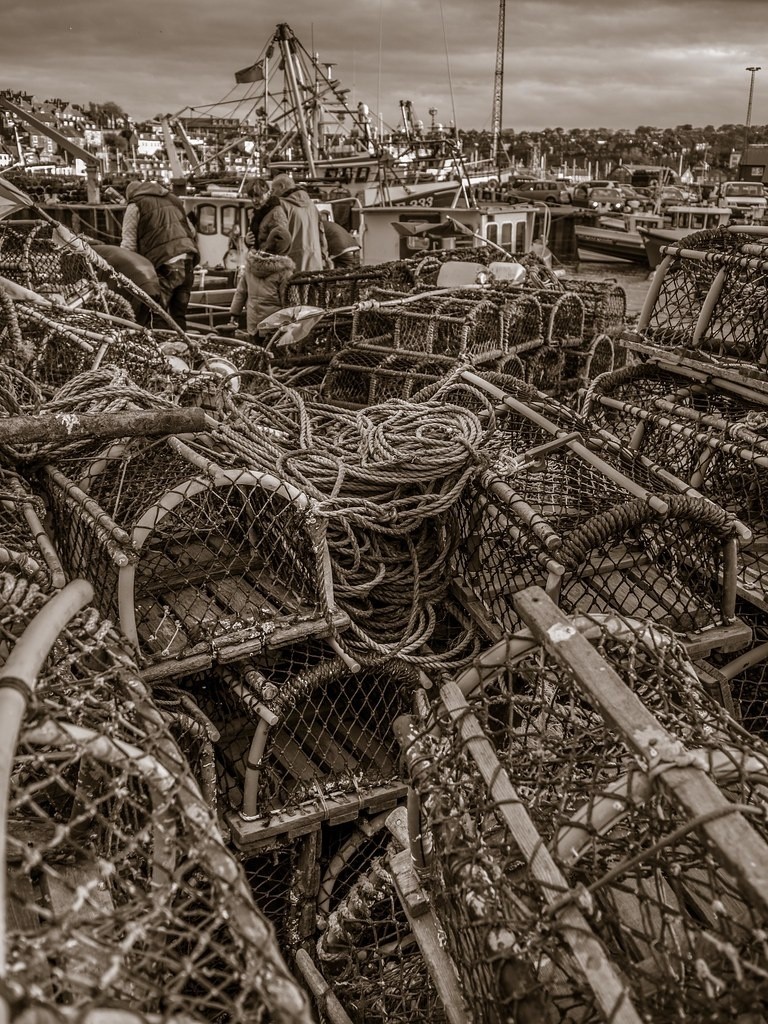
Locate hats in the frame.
[265,224,293,256]
[125,180,141,199]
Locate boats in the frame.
[0,25,553,305]
[576,206,768,268]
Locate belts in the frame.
[342,250,360,257]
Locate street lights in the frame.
[744,67,762,156]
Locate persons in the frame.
[59,233,160,327]
[120,181,201,332]
[272,173,324,306]
[231,227,301,349]
[244,178,289,251]
[322,220,361,269]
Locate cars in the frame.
[719,181,768,218]
[503,175,717,213]
